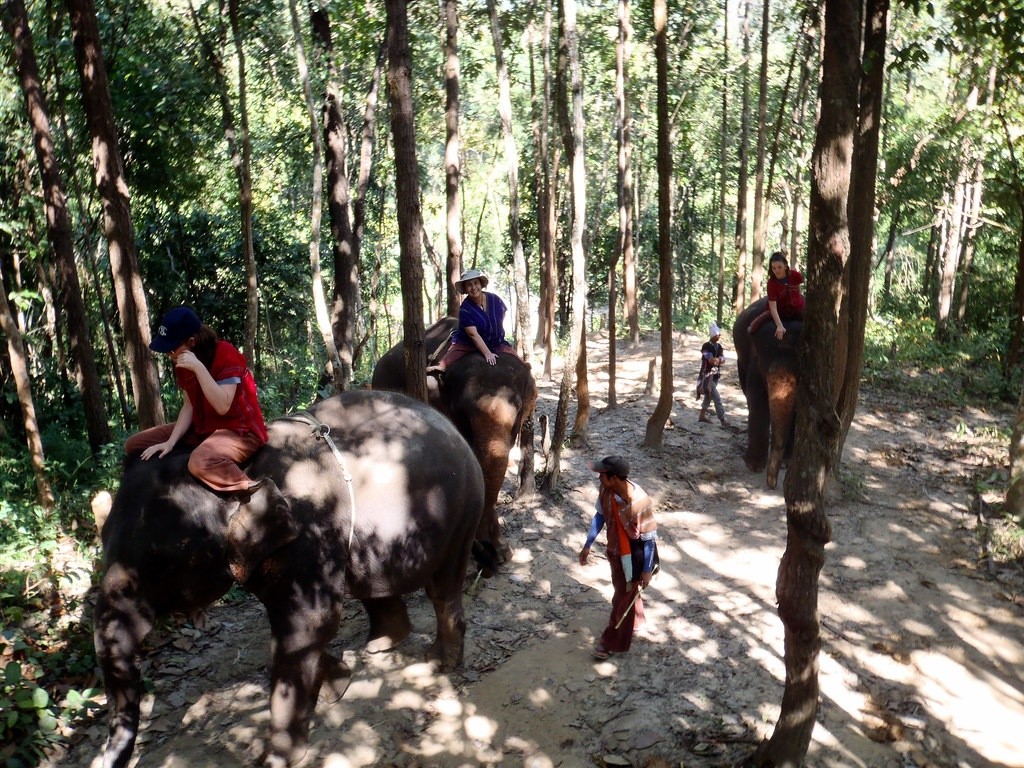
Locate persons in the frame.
[439,269,519,369]
[696,324,731,426]
[747,251,805,339]
[578,455,661,660]
[125,307,269,492]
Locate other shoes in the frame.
[698,417,712,424]
[591,644,613,659]
[722,420,730,426]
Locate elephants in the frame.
[80,385,491,768]
[368,313,542,586]
[730,289,861,495]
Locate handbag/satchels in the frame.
[697,369,714,394]
[622,538,660,582]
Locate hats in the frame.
[709,323,721,339]
[148,308,200,352]
[455,269,488,294]
[589,455,629,478]
